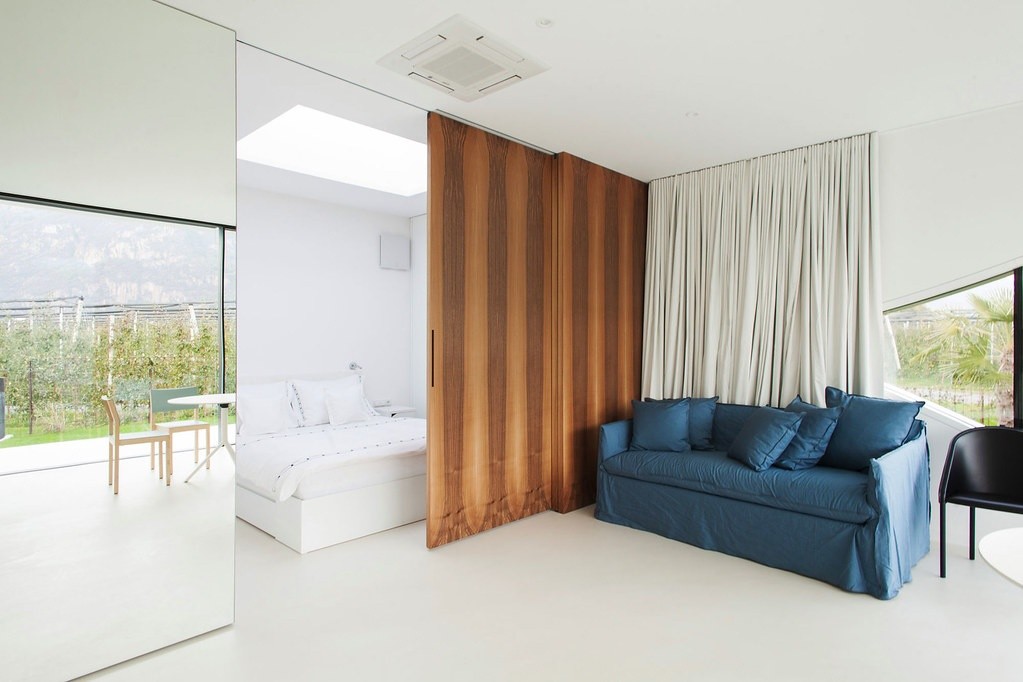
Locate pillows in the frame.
[645,396,719,451]
[712,402,762,452]
[322,381,381,426]
[774,394,844,472]
[727,404,807,472]
[628,397,691,453]
[236,381,300,436]
[286,377,381,427]
[819,386,926,473]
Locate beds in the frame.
[236,360,427,555]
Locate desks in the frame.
[167,393,236,483]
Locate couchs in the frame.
[595,402,932,600]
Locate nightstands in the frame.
[373,403,417,418]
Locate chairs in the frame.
[149,385,210,475]
[101,395,172,494]
[938,426,1023,578]
